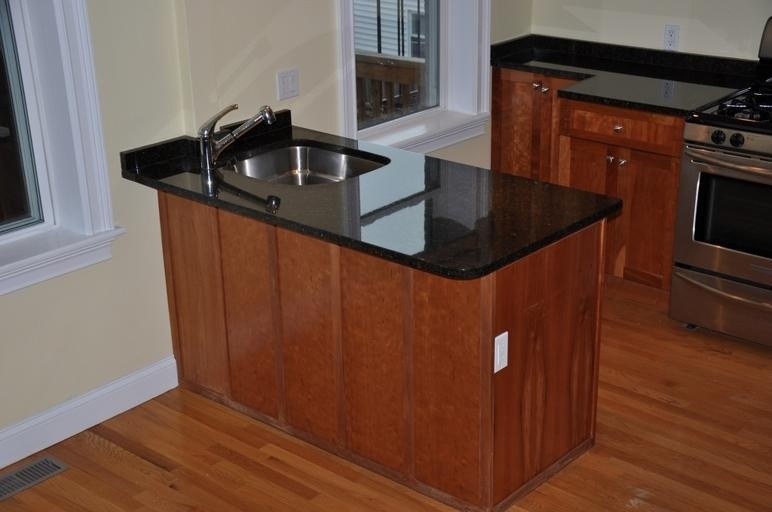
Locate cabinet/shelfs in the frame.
[491,67,582,187]
[560,98,686,291]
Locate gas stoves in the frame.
[684,68,771,154]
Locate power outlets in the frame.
[662,24,680,50]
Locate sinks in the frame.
[229,137,391,187]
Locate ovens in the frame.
[668,145,772,347]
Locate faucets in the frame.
[197,103,277,170]
[200,171,281,215]
